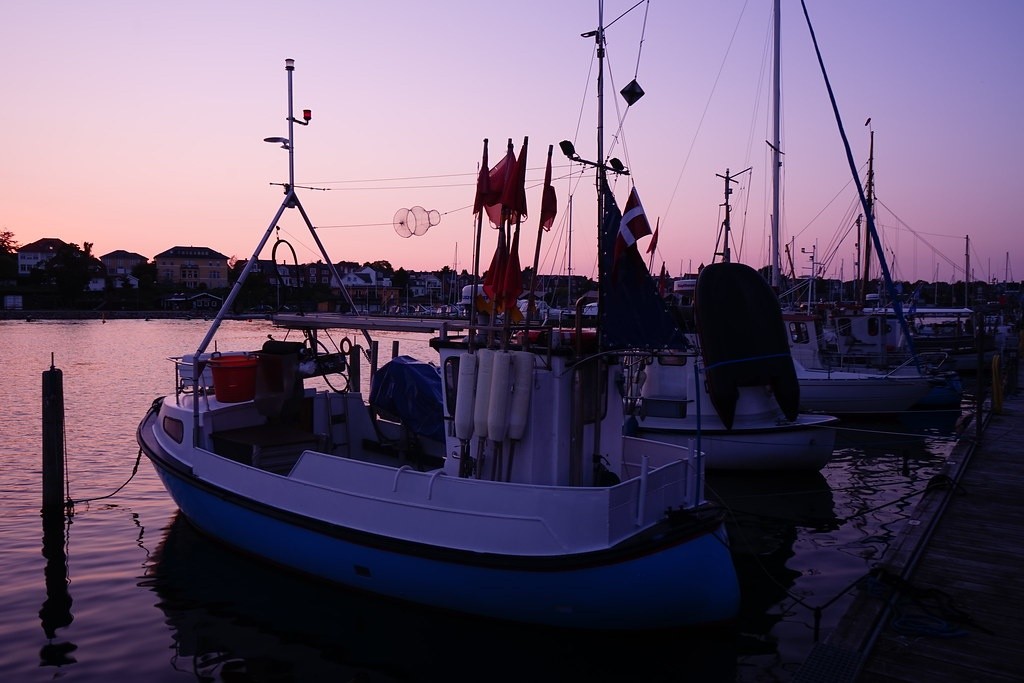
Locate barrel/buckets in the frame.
[208,352,256,403]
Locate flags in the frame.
[227,254,237,269]
[475,143,558,326]
[613,186,652,258]
[646,219,659,255]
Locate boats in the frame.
[436,0,968,474]
[135,55,746,625]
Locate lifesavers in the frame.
[991,354,1002,403]
